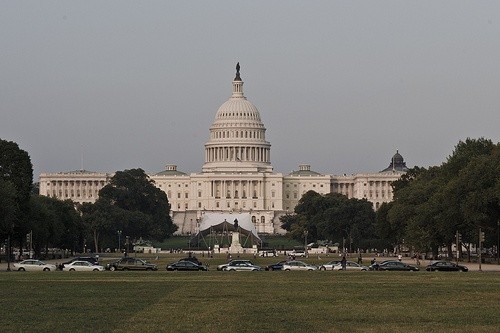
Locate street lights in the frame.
[303,230,309,252]
[117,230,122,250]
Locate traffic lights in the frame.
[26,233,31,243]
[458,233,462,243]
[4,239,8,248]
[481,232,485,242]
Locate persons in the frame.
[340,256,347,271]
[397,253,402,261]
[370,258,379,271]
[357,254,362,264]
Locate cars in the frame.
[426,260,469,272]
[287,250,306,258]
[13,248,30,256]
[58,256,420,273]
[12,259,57,272]
[259,251,278,257]
